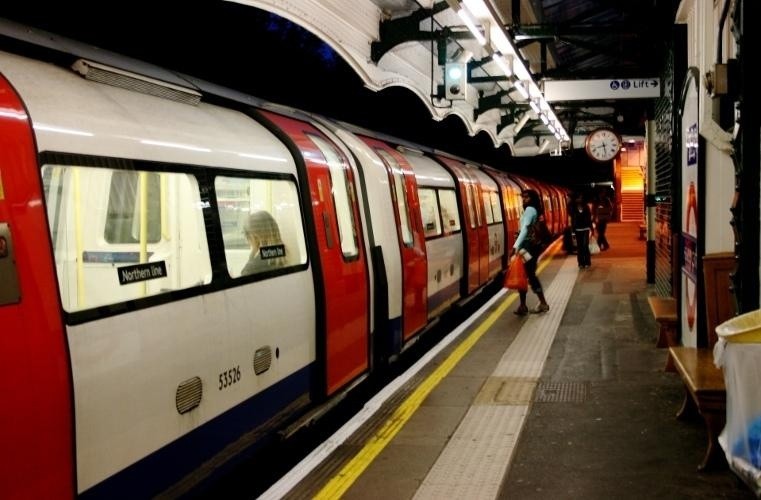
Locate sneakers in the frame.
[513,305,528,315]
[579,265,588,270]
[530,304,549,313]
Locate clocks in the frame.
[585,126,623,162]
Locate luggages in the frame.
[565,233,579,255]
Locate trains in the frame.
[1,19,574,500]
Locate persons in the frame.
[512,189,550,315]
[566,191,594,269]
[593,189,613,251]
[241,211,284,276]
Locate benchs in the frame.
[665,342,728,471]
[645,296,680,348]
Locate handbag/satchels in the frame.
[527,222,552,248]
